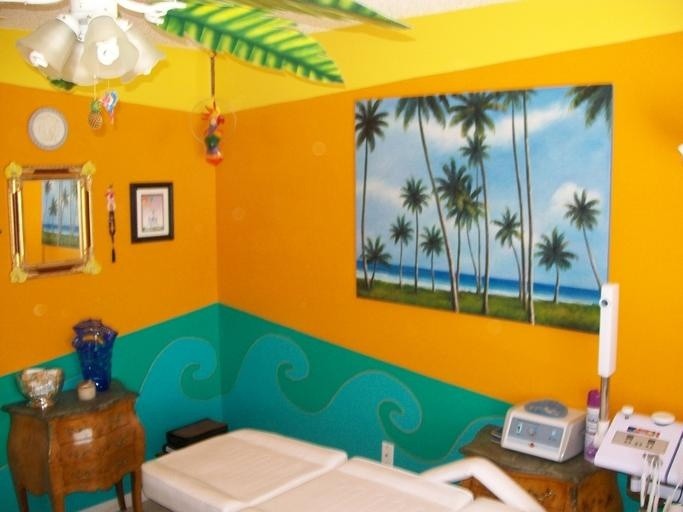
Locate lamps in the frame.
[17,1,166,88]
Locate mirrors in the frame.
[6,161,101,284]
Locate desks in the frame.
[461,423,624,512]
[0,379,146,511]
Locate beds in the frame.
[140,426,551,512]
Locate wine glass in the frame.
[15,367,67,411]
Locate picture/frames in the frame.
[128,182,175,242]
